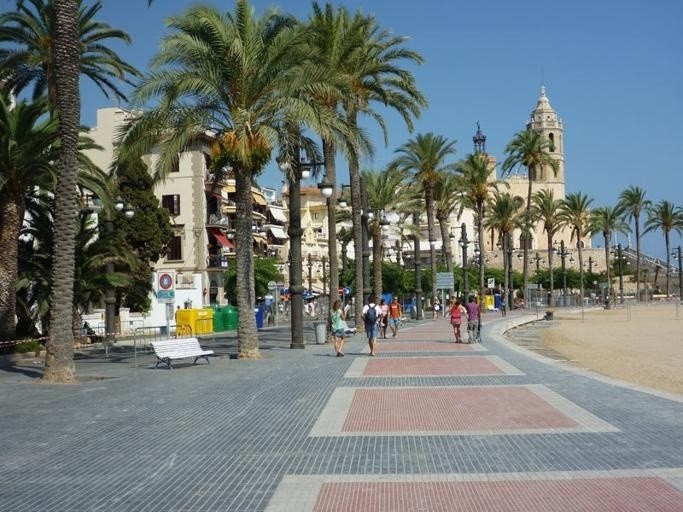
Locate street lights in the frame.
[449,223,481,309]
[582,256,599,288]
[495,235,517,310]
[551,240,575,296]
[275,154,335,350]
[609,243,632,305]
[386,239,412,265]
[530,250,546,290]
[671,245,683,302]
[337,176,372,305]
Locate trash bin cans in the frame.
[176,305,238,336]
[256,306,263,328]
[478,294,500,308]
[313,322,327,345]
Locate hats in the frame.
[394,297,397,300]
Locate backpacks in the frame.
[365,304,376,324]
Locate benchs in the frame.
[150,338,214,370]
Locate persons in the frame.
[389,296,402,338]
[500,293,507,317]
[377,298,390,339]
[264,299,314,322]
[431,296,441,320]
[328,299,348,356]
[360,295,384,356]
[464,296,481,344]
[447,296,469,343]
[448,295,457,315]
